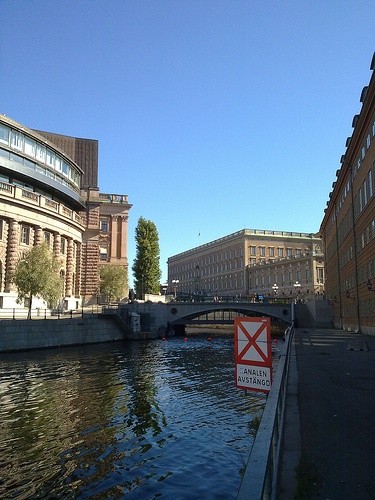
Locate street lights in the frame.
[272,284,279,303]
[293,281,302,304]
[171,279,179,298]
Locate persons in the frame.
[255,293,259,301]
